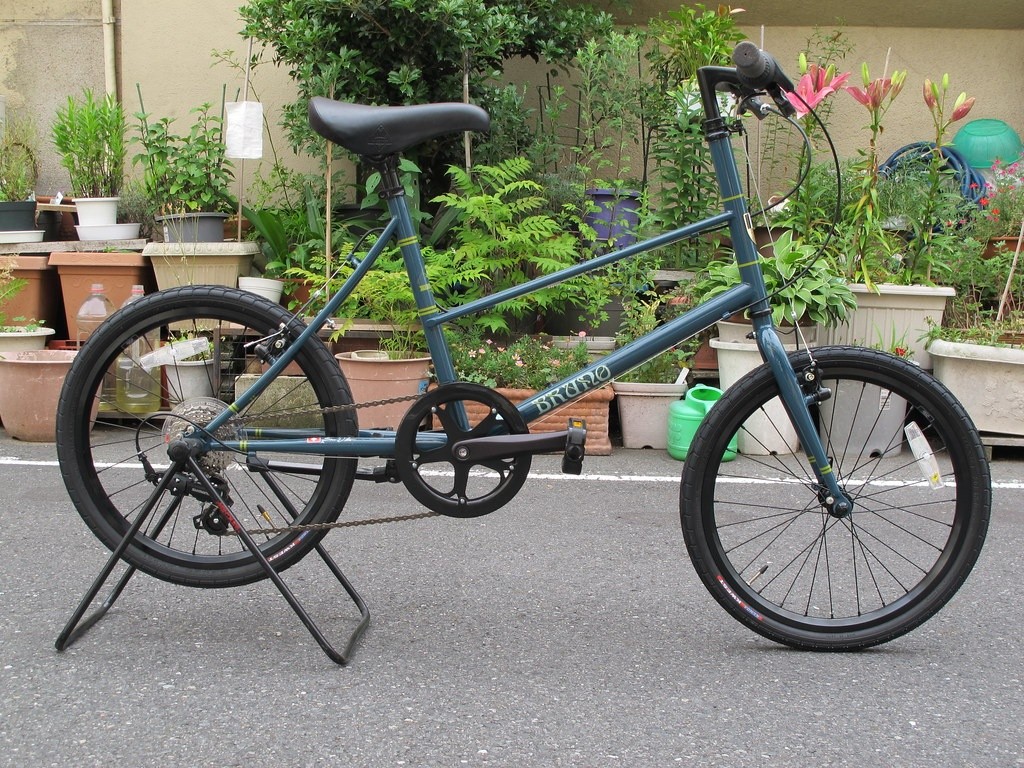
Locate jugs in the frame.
[667,383,738,462]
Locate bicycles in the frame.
[50,40,996,669]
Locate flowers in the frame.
[840,322,916,362]
[786,47,976,296]
[450,333,598,388]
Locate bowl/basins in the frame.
[949,119,1024,168]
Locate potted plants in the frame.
[1,1,1024,462]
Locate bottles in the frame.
[115,285,161,412]
[76,284,116,411]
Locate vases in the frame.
[141,240,284,323]
[0,256,49,326]
[426,379,613,455]
[820,379,906,457]
[1,349,102,442]
[817,283,955,369]
[708,337,816,456]
